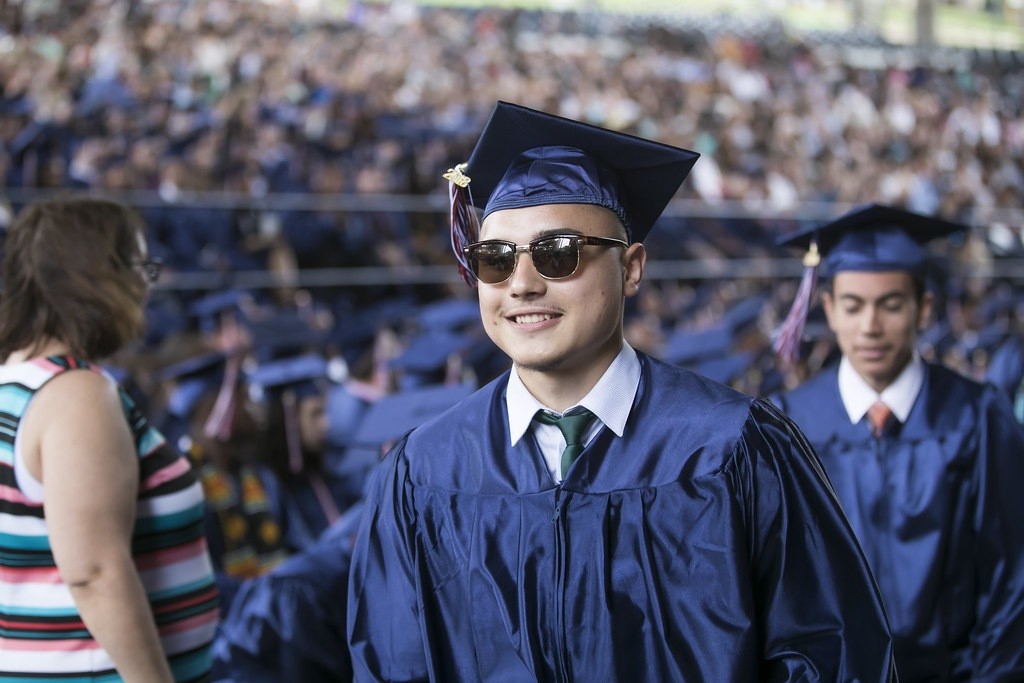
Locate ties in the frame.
[866,404,894,439]
[532,410,595,480]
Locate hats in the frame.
[442,100,700,288]
[770,201,973,362]
[665,302,763,388]
[173,292,479,476]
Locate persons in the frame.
[346,96,899,683]
[0,199,221,683]
[0,0,1024,552]
[739,199,1024,683]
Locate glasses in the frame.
[463,234,629,284]
[146,256,163,279]
